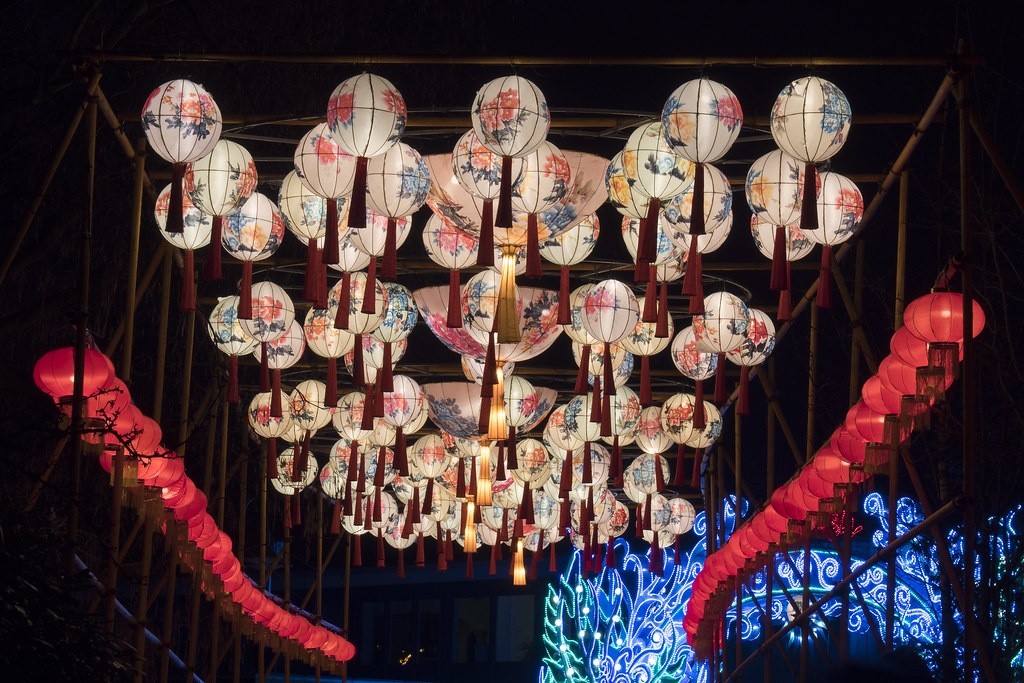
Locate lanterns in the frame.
[140,74,864,585]
[34,345,356,672]
[683,293,984,663]
